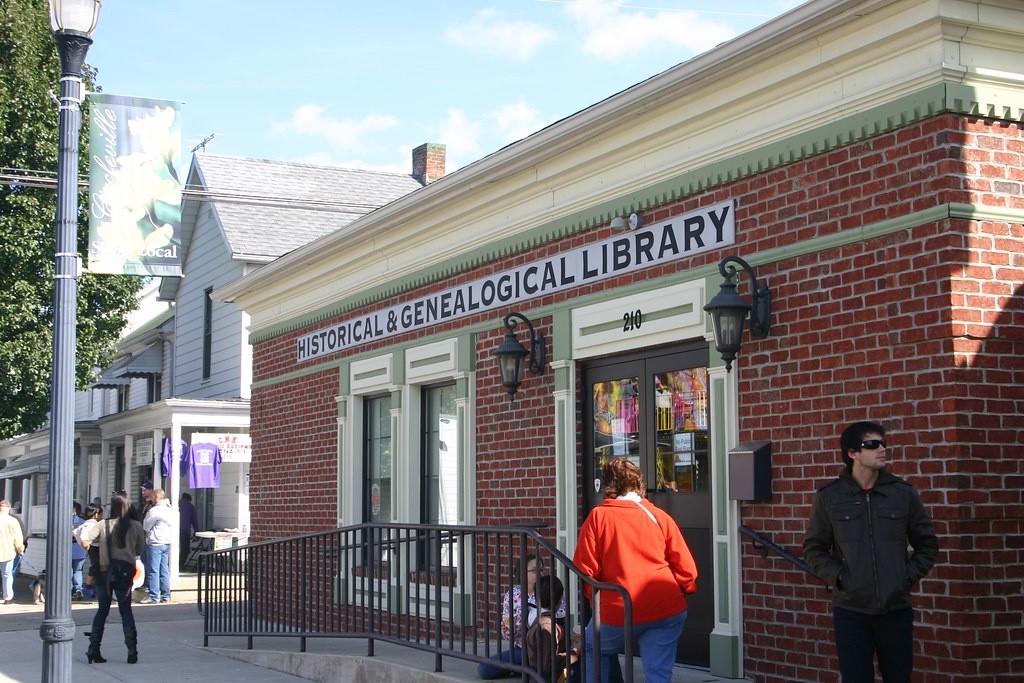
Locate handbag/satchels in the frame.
[107,559,134,602]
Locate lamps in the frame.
[610,213,644,231]
[702,256,772,372]
[492,313,545,401]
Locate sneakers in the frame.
[140,595,159,604]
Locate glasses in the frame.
[851,438,887,449]
[526,568,547,574]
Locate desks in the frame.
[184,532,238,573]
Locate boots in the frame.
[123,625,138,664]
[82,627,108,664]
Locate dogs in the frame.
[31,575,45,605]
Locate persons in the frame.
[137,482,152,523]
[83,491,145,664]
[572,458,698,683]
[476,554,566,680]
[803,422,939,683]
[0,500,24,604]
[179,493,198,571]
[72,503,103,600]
[141,489,172,603]
[526,575,582,683]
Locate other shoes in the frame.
[3,596,17,604]
[33,593,45,605]
[76,589,83,600]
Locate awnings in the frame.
[87,341,162,415]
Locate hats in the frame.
[0,499,11,508]
[140,481,153,490]
